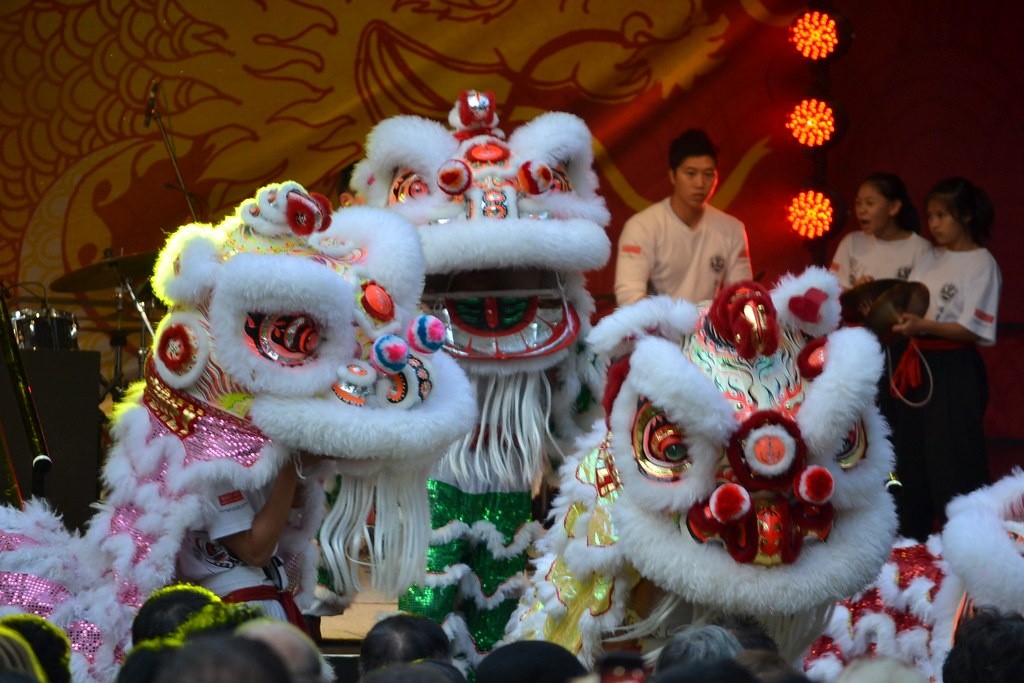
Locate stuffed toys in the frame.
[800,467,1024,683]
[313,90,610,646]
[501,264,900,664]
[0,183,476,683]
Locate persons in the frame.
[337,163,365,207]
[830,172,931,292]
[175,450,333,634]
[616,127,751,309]
[894,178,1001,540]
[0,582,1024,683]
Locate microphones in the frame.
[144,78,158,126]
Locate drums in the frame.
[0,309,81,352]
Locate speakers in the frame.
[0,347,101,542]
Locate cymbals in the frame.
[50,249,160,292]
[839,277,930,343]
